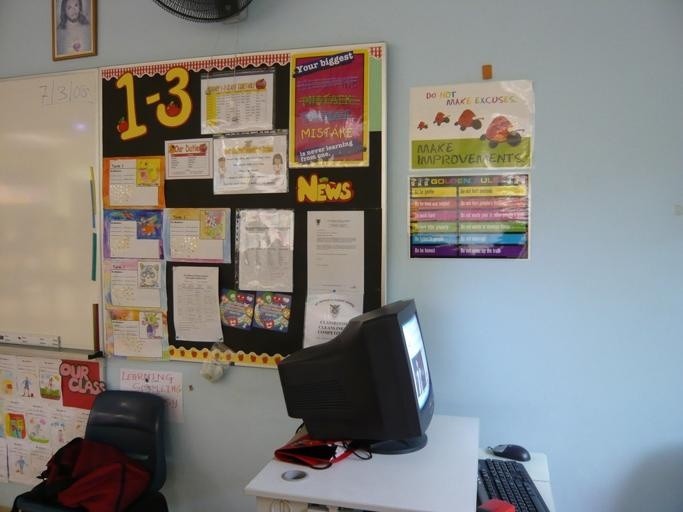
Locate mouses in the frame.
[492,443,531,463]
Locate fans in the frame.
[153,0,252,25]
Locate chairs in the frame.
[14,390,169,511]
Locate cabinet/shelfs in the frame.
[242,414,554,511]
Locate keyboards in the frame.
[475,458,551,512]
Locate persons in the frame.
[217,157,227,177]
[272,153,284,176]
[56,0,92,56]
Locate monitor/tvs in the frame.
[277,299,436,455]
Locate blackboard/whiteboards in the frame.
[0,68,102,355]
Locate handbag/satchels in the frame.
[38,437,148,512]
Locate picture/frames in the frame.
[51,1,98,61]
